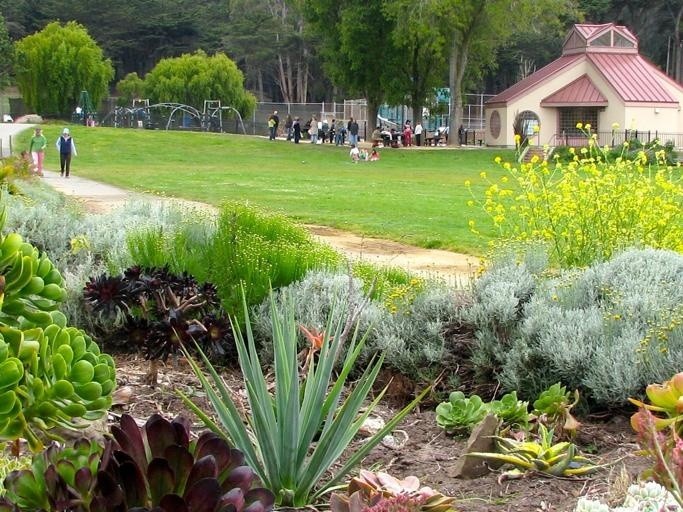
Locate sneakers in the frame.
[60,173,70,178]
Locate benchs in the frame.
[373,130,484,148]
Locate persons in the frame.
[56,128,77,177]
[367,149,380,162]
[358,149,368,161]
[266,111,465,148]
[29,127,46,172]
[349,145,359,162]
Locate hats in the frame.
[63,128,69,134]
